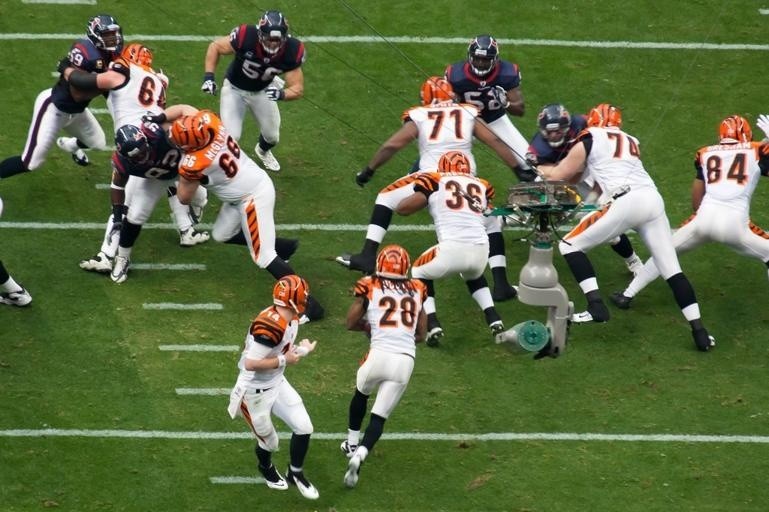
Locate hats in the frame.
[256,9,289,53]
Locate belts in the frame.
[256,389,269,393]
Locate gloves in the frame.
[491,84,511,108]
[107,222,123,246]
[356,167,374,188]
[202,79,217,96]
[756,115,769,140]
[265,86,281,101]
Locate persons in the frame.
[340,244,428,488]
[105,104,325,325]
[203,9,306,171]
[227,275,321,499]
[610,114,769,309]
[1,263,32,307]
[1,14,210,271]
[335,33,716,350]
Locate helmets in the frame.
[536,103,571,149]
[121,42,153,71]
[718,114,753,143]
[438,152,470,175]
[114,124,150,165]
[172,113,209,149]
[586,103,621,128]
[86,13,124,57]
[419,77,457,107]
[466,34,499,78]
[374,244,412,280]
[271,275,310,316]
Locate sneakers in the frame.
[180,227,210,246]
[334,254,375,277]
[423,327,445,346]
[80,252,113,273]
[258,440,363,501]
[624,251,646,278]
[494,284,518,302]
[697,334,716,353]
[254,142,281,171]
[570,304,610,325]
[0,286,32,307]
[609,289,634,310]
[491,323,506,344]
[54,136,89,167]
[282,238,299,263]
[110,256,130,283]
